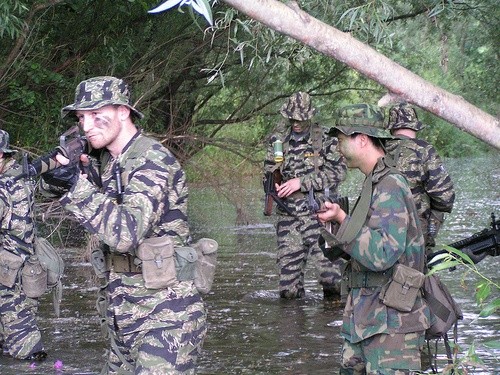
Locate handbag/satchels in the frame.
[88,238,108,278]
[424,271,463,340]
[34,236,64,288]
[379,264,425,313]
[187,238,218,295]
[0,250,23,288]
[136,236,176,290]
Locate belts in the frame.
[289,191,306,198]
[105,253,177,271]
[348,272,388,287]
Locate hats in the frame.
[0,129,18,152]
[61,75,145,119]
[390,108,423,131]
[279,91,316,122]
[324,104,397,140]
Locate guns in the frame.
[263,159,292,218]
[425,213,500,272]
[288,178,350,224]
[6,125,92,186]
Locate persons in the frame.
[39,76,207,375]
[312,104,436,375]
[263,93,347,298]
[0,130,46,359]
[385,106,456,366]
